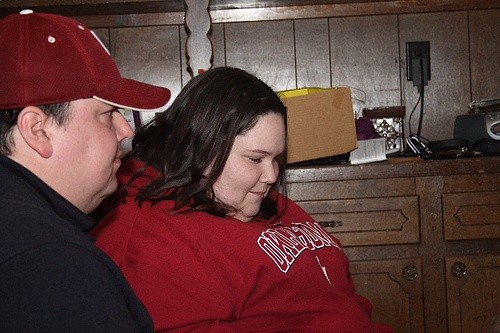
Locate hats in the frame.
[0,9,171,110]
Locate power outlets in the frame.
[406,41,431,81]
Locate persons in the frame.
[0,10,172,333]
[87,67,394,333]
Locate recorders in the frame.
[453,111,500,156]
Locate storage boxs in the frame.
[274,86,358,165]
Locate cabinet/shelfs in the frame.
[274,155,500,333]
